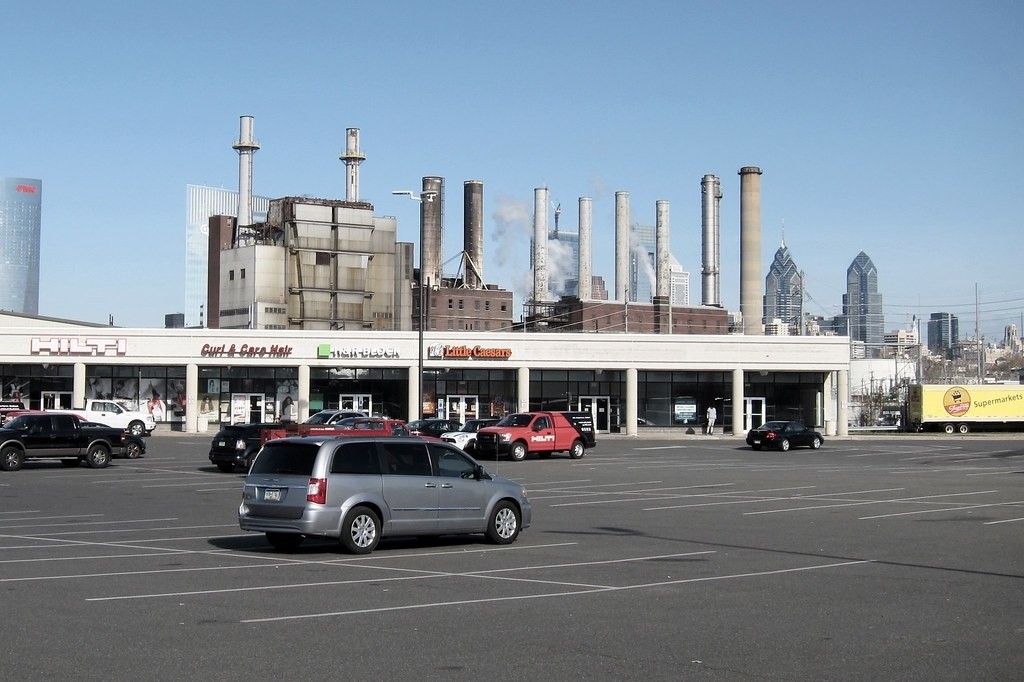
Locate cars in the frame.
[439,419,503,455]
[78,421,147,459]
[206,423,290,473]
[331,416,372,430]
[4,411,89,426]
[391,418,464,441]
[745,420,825,451]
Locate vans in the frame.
[0,401,25,427]
[238,437,534,557]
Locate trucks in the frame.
[258,418,443,448]
[908,383,1024,435]
[476,410,598,460]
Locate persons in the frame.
[705,403,717,435]
[150,388,162,409]
[113,380,125,398]
[174,383,186,414]
[200,395,214,415]
[89,378,108,397]
[7,383,24,400]
[282,396,296,416]
[208,380,216,392]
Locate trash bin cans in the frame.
[197,414,209,432]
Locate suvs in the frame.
[298,408,369,427]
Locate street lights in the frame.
[390,188,439,421]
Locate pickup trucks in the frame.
[44,398,157,437]
[0,414,128,472]
